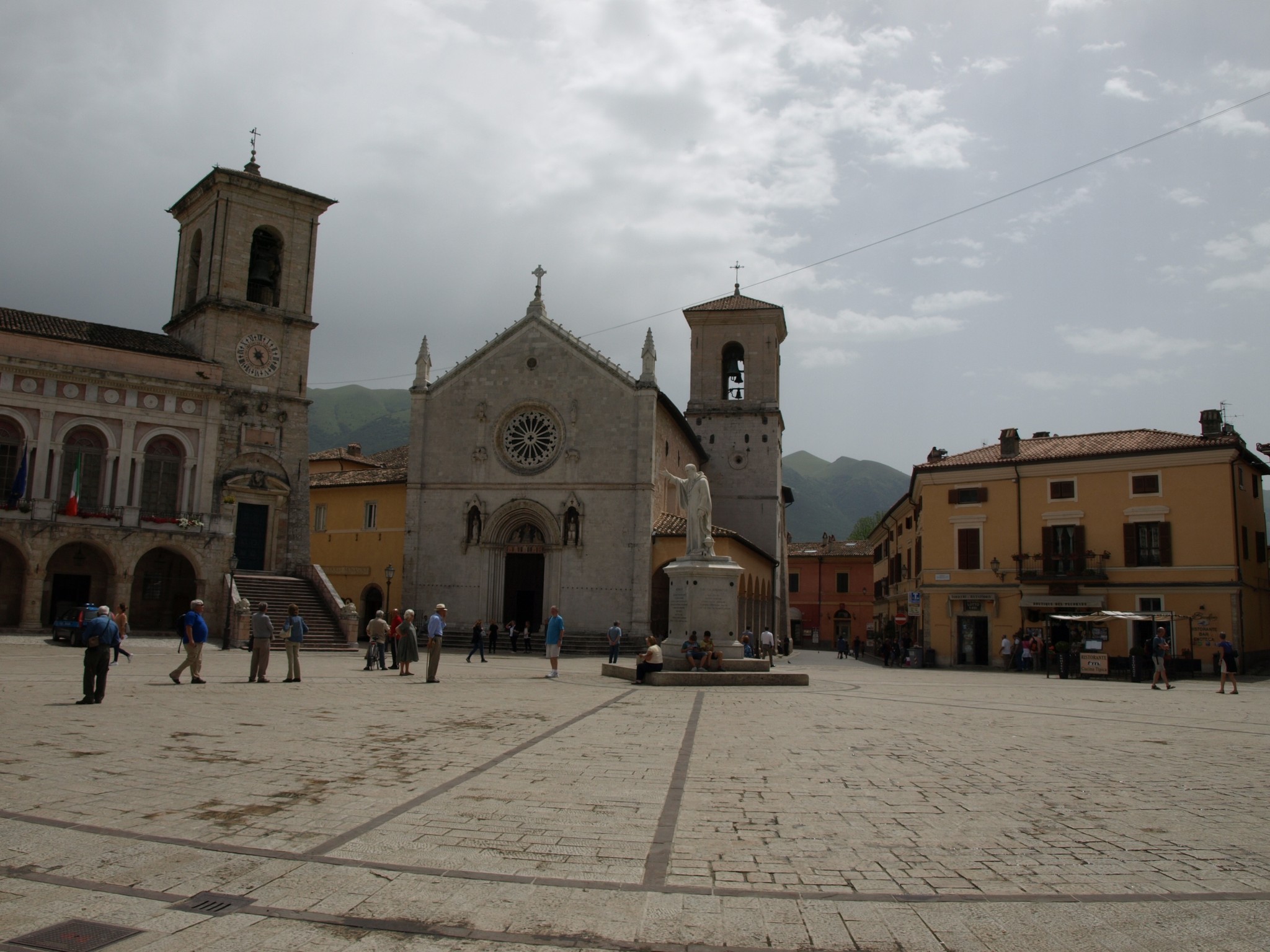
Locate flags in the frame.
[65,452,80,516]
[8,444,27,508]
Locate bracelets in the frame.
[558,639,561,640]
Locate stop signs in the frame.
[895,613,907,625]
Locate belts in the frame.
[434,634,441,637]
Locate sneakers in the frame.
[691,666,727,672]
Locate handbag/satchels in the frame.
[1031,637,1038,652]
[88,635,99,648]
[279,618,293,638]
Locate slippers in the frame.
[631,680,641,685]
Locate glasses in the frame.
[198,604,204,607]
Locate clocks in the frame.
[236,332,282,378]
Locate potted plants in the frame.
[1018,569,1108,580]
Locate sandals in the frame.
[1167,685,1176,690]
[1152,685,1160,690]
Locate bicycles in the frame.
[368,636,381,671]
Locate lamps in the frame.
[863,587,866,596]
[238,406,247,417]
[880,577,898,591]
[852,613,856,620]
[826,612,830,620]
[259,401,268,413]
[900,565,921,581]
[278,411,288,423]
[990,557,1005,583]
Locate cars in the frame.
[52,606,115,647]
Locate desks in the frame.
[1108,657,1202,679]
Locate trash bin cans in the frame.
[907,647,923,668]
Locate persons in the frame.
[76,605,120,705]
[169,599,208,684]
[999,632,1044,672]
[111,603,130,663]
[544,605,564,677]
[427,604,447,683]
[741,626,790,667]
[1216,631,1238,694]
[466,620,487,662]
[607,620,622,663]
[488,619,532,653]
[631,636,663,684]
[423,615,429,633]
[363,608,419,676]
[1151,627,1175,689]
[249,602,273,682]
[837,635,865,660]
[282,604,308,682]
[880,632,913,668]
[681,631,727,672]
[658,464,716,558]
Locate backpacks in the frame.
[175,613,198,638]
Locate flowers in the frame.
[1012,550,1111,557]
[0,504,204,530]
[224,496,236,504]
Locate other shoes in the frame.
[169,673,180,684]
[545,672,558,678]
[283,678,293,682]
[110,660,117,664]
[76,697,95,705]
[481,659,488,663]
[1216,691,1224,693]
[293,678,301,682]
[364,664,441,683]
[191,677,206,684]
[127,654,134,662]
[770,665,775,667]
[249,679,256,682]
[1230,691,1239,693]
[257,679,270,683]
[466,657,471,662]
[94,696,101,704]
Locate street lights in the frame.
[384,563,395,652]
[222,553,239,651]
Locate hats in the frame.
[434,603,448,610]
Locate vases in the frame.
[1085,554,1096,558]
[1052,556,1062,560]
[1036,556,1046,560]
[1067,556,1079,560]
[223,503,237,509]
[1104,555,1110,559]
[1013,556,1029,561]
